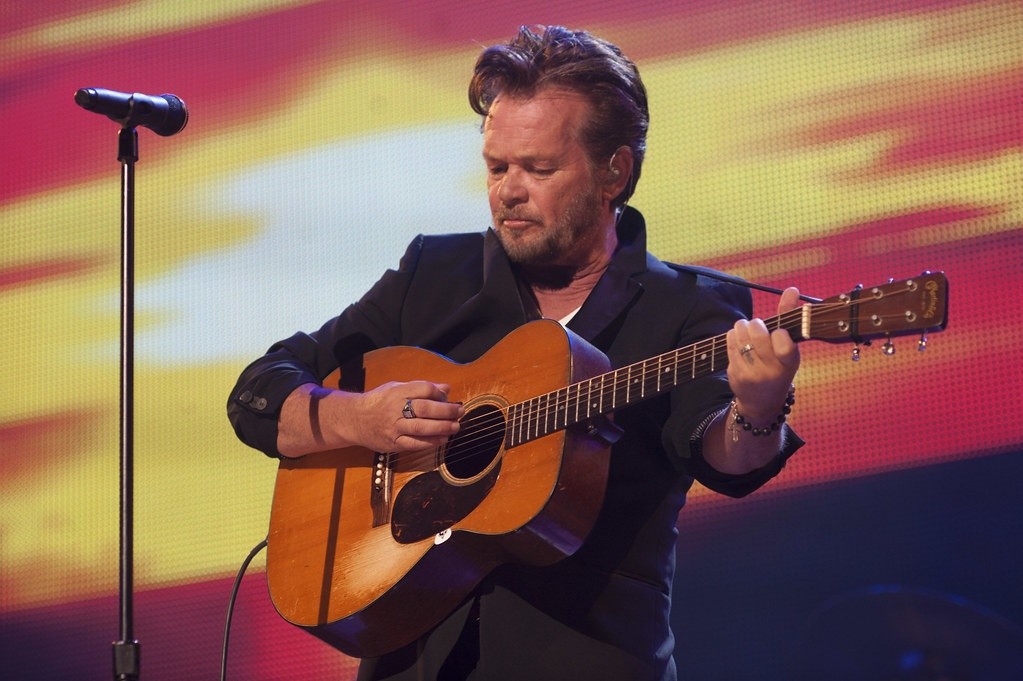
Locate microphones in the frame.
[74,87,189,137]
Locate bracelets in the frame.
[730,384,796,436]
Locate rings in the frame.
[402,399,417,418]
[740,343,754,355]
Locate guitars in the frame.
[266,270,950,658]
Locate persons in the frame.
[226,24,801,680]
